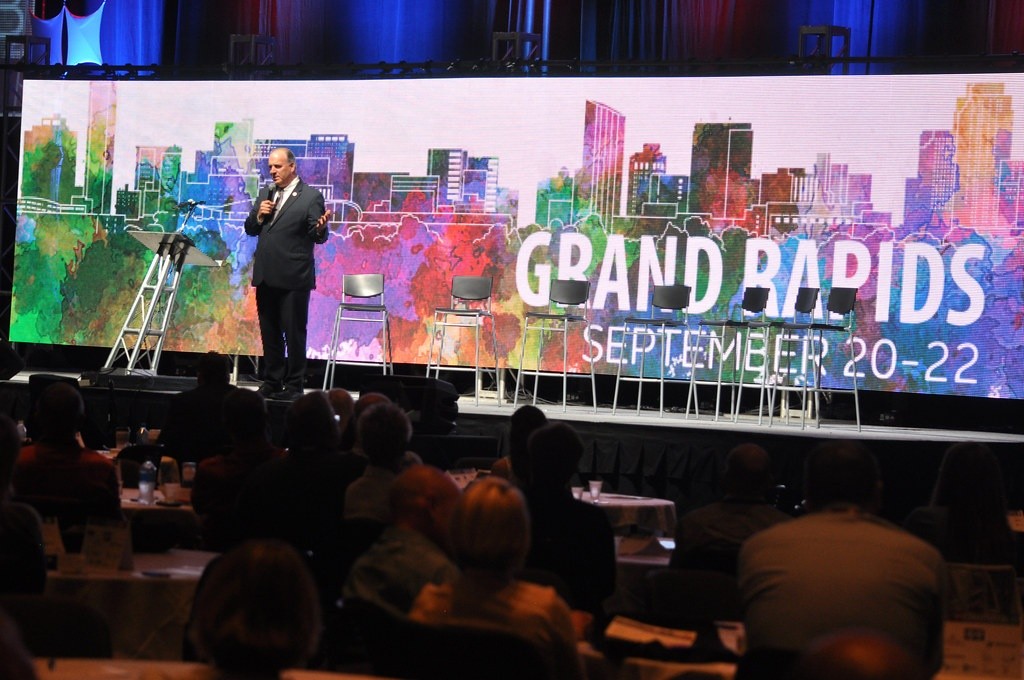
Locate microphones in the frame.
[263,183,276,218]
[177,199,194,208]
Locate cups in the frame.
[571,486,584,500]
[114,431,130,450]
[588,481,603,502]
[110,449,122,457]
[149,429,161,444]
[131,460,180,506]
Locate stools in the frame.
[426,274,502,408]
[513,278,597,416]
[685,287,861,433]
[612,285,700,420]
[323,274,394,392]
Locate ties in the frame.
[267,187,284,228]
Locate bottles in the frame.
[16,420,27,442]
[181,462,195,488]
[135,423,149,445]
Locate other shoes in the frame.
[256,384,268,398]
[269,388,304,400]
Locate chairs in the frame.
[11,494,88,553]
[115,444,175,489]
[370,609,560,680]
[129,509,199,554]
[0,591,113,657]
[640,568,742,623]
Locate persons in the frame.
[791,627,921,679]
[907,442,1019,575]
[735,443,942,680]
[669,443,796,660]
[188,539,319,679]
[244,148,333,398]
[0,353,616,680]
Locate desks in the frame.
[46,554,220,660]
[33,658,383,680]
[581,491,676,555]
[120,487,206,529]
